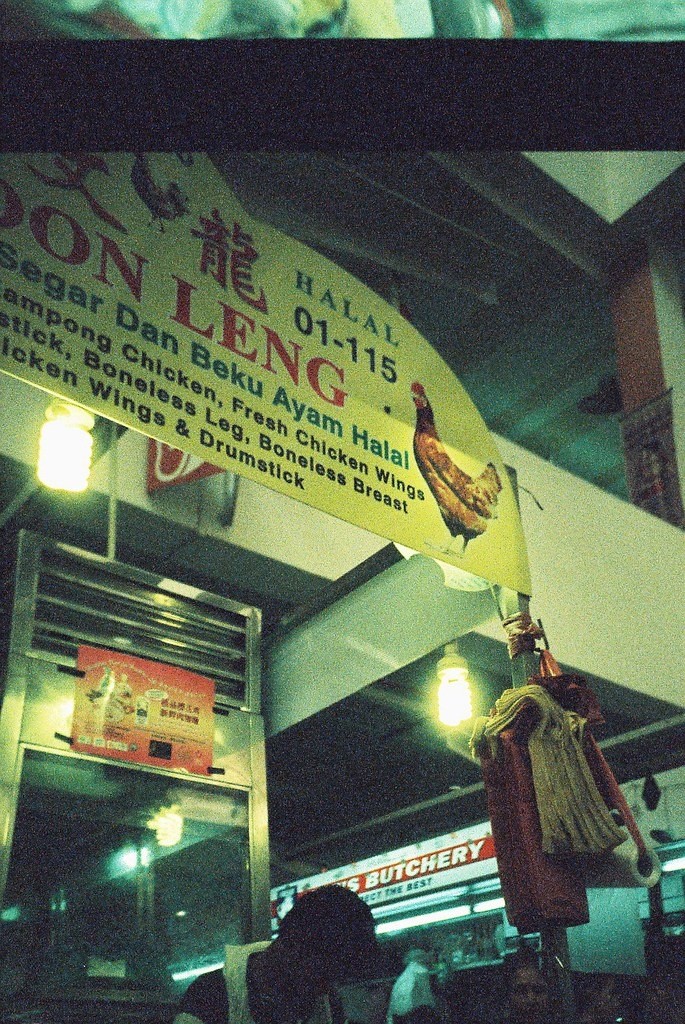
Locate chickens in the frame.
[407,380,503,558]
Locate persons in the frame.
[575,972,621,1024]
[171,884,381,1024]
[500,951,549,1024]
[386,949,450,1024]
